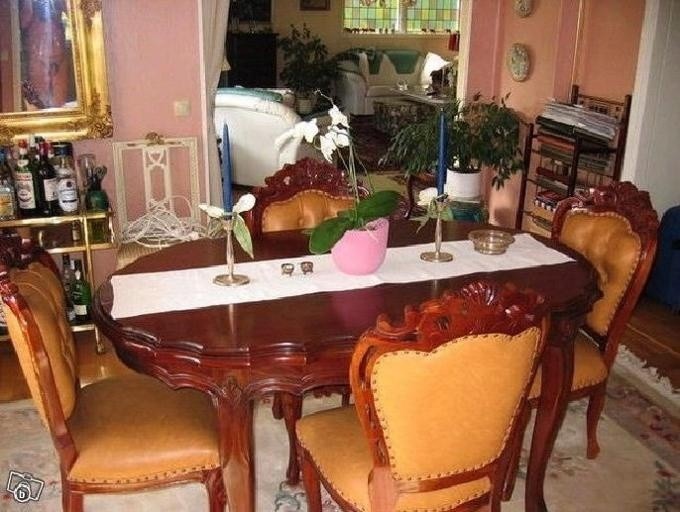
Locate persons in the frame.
[17,0,73,112]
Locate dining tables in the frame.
[275,23,343,113]
[387,89,524,204]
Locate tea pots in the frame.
[0,0,114,147]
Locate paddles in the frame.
[0,340,680,512]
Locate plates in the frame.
[516,87,634,240]
[227,32,283,86]
[1,208,118,354]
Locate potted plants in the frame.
[299,0,331,11]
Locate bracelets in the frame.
[467,229,515,254]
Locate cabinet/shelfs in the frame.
[0,176,15,220]
[71,259,91,322]
[62,253,76,303]
[79,155,95,185]
[83,173,110,242]
[36,141,58,217]
[0,149,12,182]
[51,141,79,218]
[32,136,45,171]
[14,140,39,217]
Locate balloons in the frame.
[507,42,531,81]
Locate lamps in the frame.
[495,182,661,503]
[245,155,368,419]
[111,131,223,271]
[1,257,226,510]
[295,276,556,511]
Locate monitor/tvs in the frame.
[525,95,619,237]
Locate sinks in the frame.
[213,87,343,190]
[335,48,454,117]
